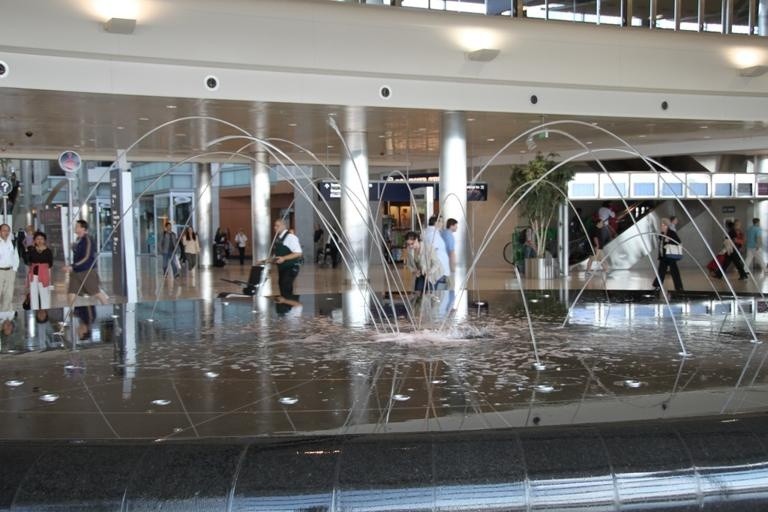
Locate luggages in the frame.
[705,252,728,272]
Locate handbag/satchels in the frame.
[663,242,685,259]
[22,293,41,311]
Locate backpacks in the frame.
[518,227,533,244]
[275,231,295,273]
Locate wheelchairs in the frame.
[218,259,277,299]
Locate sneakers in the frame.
[586,269,615,277]
[654,287,661,291]
[712,273,749,280]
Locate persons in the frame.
[384,290,456,325]
[666,216,679,273]
[1,219,111,308]
[381,201,458,290]
[647,218,686,298]
[0,305,98,342]
[271,295,304,321]
[160,216,340,324]
[519,200,627,279]
[710,218,768,282]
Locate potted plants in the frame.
[505,146,581,281]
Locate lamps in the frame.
[101,18,140,38]
[466,46,501,65]
[739,64,767,82]
